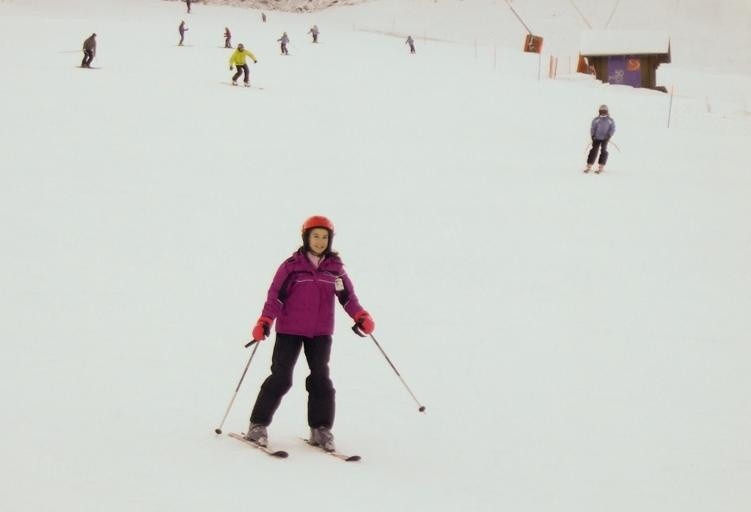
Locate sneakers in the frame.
[311,425,333,450]
[249,421,268,446]
[233,81,238,86]
[245,81,250,87]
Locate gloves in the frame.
[254,317,272,341]
[354,308,374,334]
[604,135,610,144]
[593,135,596,142]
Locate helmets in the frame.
[302,216,333,245]
[598,106,607,117]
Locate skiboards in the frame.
[73,66,100,69]
[584,169,601,174]
[228,432,361,462]
[219,82,264,90]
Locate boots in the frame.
[583,163,591,172]
[596,164,603,173]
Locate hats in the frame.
[238,44,242,48]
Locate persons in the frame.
[406,36,416,54]
[178,20,189,46]
[307,24,321,43]
[277,32,291,55]
[81,32,98,68]
[585,104,618,172]
[224,27,232,48]
[228,43,258,87]
[187,1,192,14]
[246,214,377,452]
